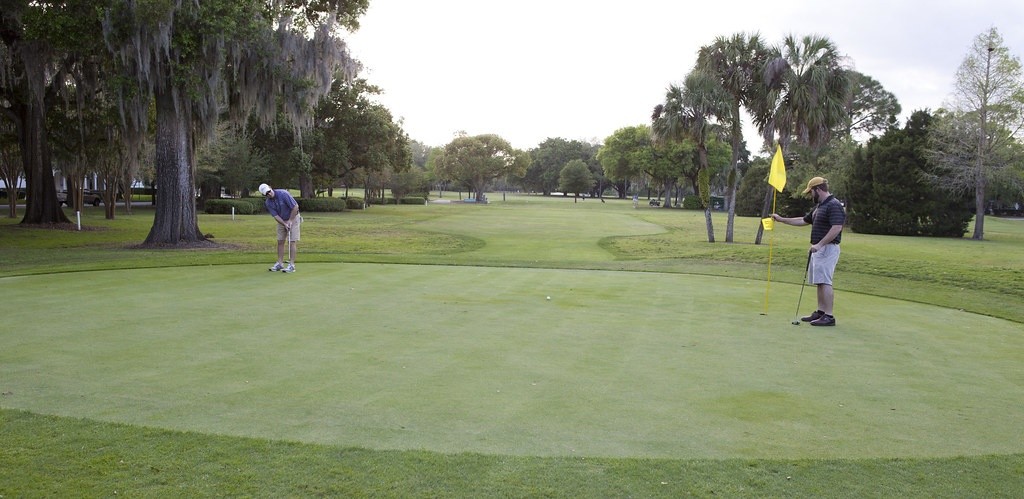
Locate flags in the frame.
[768,145,787,193]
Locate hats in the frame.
[803,177,829,194]
[259,184,272,196]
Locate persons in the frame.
[770,177,845,326]
[633,193,638,207]
[259,183,300,273]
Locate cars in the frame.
[57,188,102,208]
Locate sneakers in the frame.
[269,262,284,272]
[810,315,835,326]
[281,264,296,273]
[801,311,822,322]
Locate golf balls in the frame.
[546,296,551,300]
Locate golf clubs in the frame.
[791,250,812,325]
[282,223,291,273]
